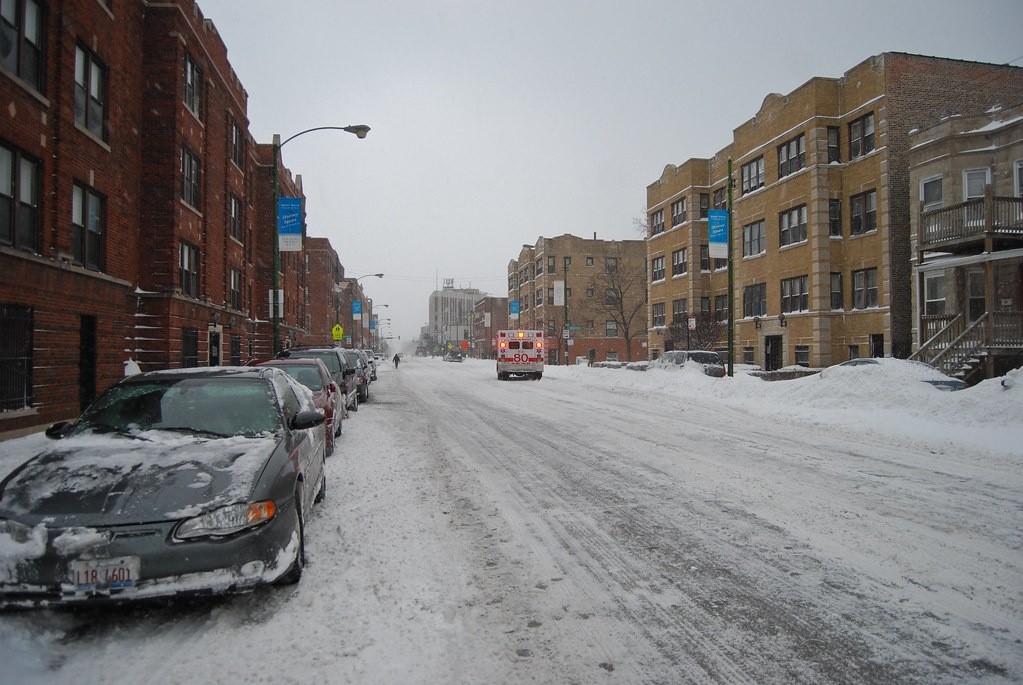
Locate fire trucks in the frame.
[496,330,545,381]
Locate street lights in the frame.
[369,305,391,352]
[272,125,370,354]
[351,273,384,348]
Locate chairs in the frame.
[296,370,319,390]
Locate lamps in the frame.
[208,312,221,327]
[223,317,236,329]
[753,317,761,329]
[778,313,786,327]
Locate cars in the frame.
[0,365,331,617]
[647,350,726,378]
[262,345,379,460]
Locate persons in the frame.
[393,354,400,369]
